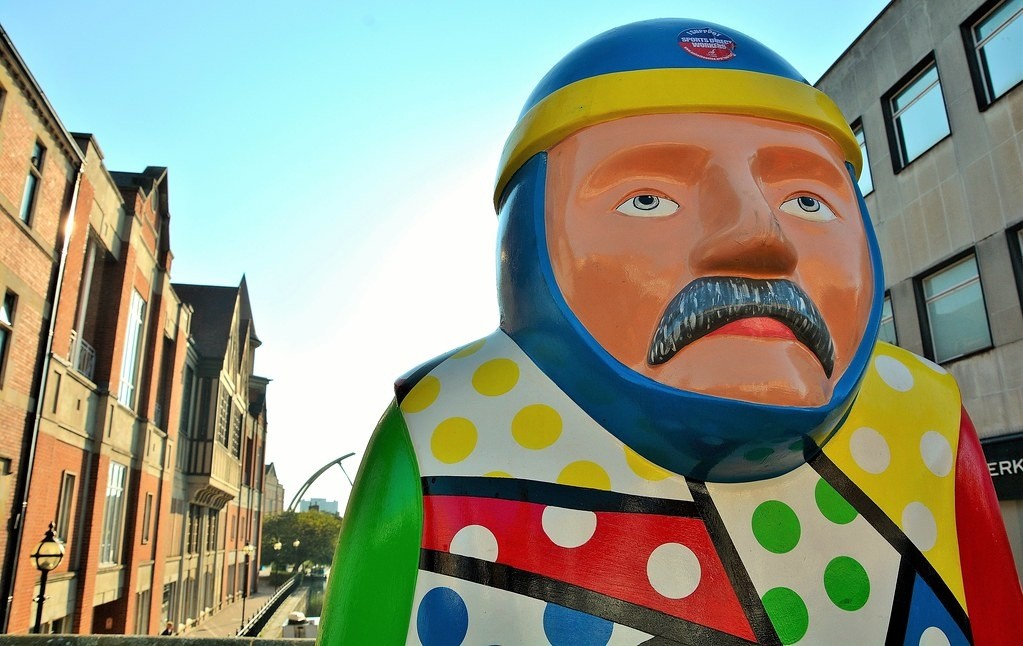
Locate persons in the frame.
[161,622,173,636]
[317,18,1023,645]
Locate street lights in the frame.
[292,537,300,573]
[30,522,66,634]
[273,538,282,593]
[240,542,253,635]
